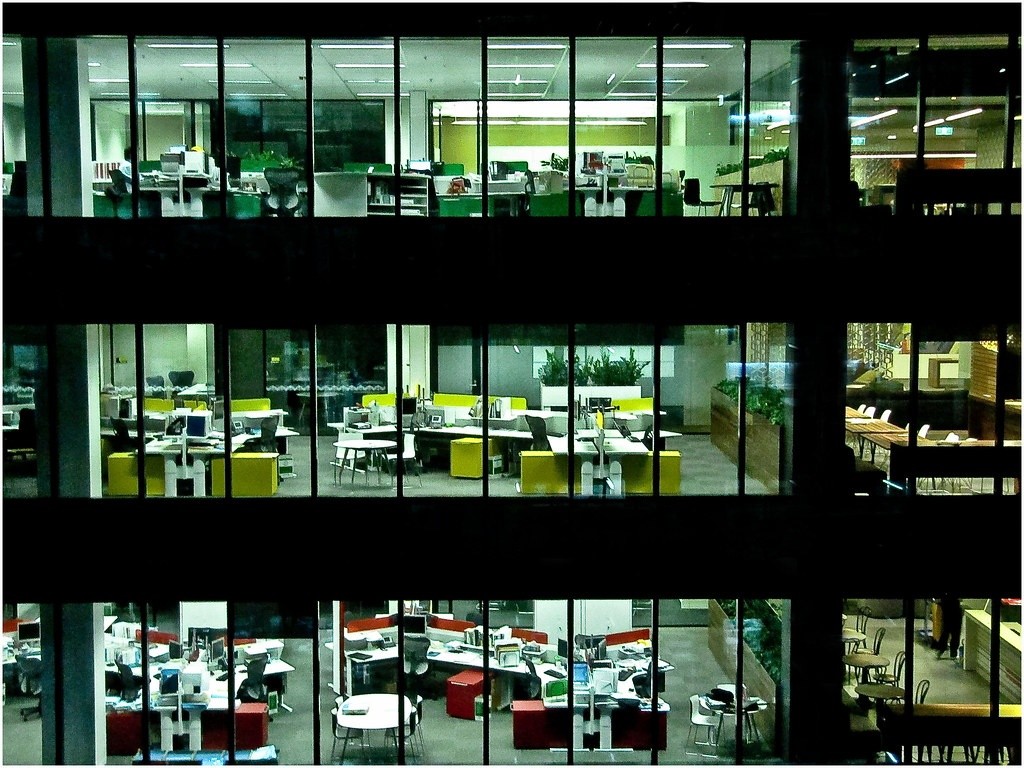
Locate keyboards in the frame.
[618,669,632,681]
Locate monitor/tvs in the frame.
[404,615,426,636]
[574,399,581,422]
[211,637,225,662]
[169,639,182,659]
[17,621,41,642]
[557,638,568,660]
[403,397,417,416]
[213,400,224,421]
[598,638,607,660]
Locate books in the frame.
[464,625,496,647]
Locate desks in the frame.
[933,439,1020,493]
[859,433,937,463]
[845,418,908,455]
[701,696,767,748]
[856,683,905,706]
[843,629,866,655]
[4,426,19,431]
[845,406,871,419]
[331,439,396,489]
[141,169,208,217]
[104,383,299,495]
[927,358,960,388]
[326,625,676,752]
[3,632,42,665]
[888,704,1022,765]
[103,613,295,714]
[843,655,890,686]
[326,409,683,497]
[337,694,414,766]
[435,185,653,218]
[710,184,756,216]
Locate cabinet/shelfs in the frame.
[316,171,431,218]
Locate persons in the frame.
[934,597,964,661]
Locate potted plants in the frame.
[704,600,784,751]
[541,154,570,177]
[709,377,780,490]
[712,146,788,215]
[535,348,650,410]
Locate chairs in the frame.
[465,612,485,625]
[643,424,654,448]
[15,655,43,721]
[102,153,303,219]
[147,376,166,388]
[840,607,931,728]
[844,404,1022,496]
[235,660,268,704]
[401,635,438,700]
[109,416,138,454]
[384,433,424,489]
[523,657,543,698]
[751,181,776,216]
[849,181,864,205]
[524,414,554,452]
[115,659,139,701]
[251,416,286,484]
[686,684,752,758]
[684,178,725,215]
[7,409,37,463]
[169,371,195,388]
[635,660,651,698]
[330,693,424,761]
